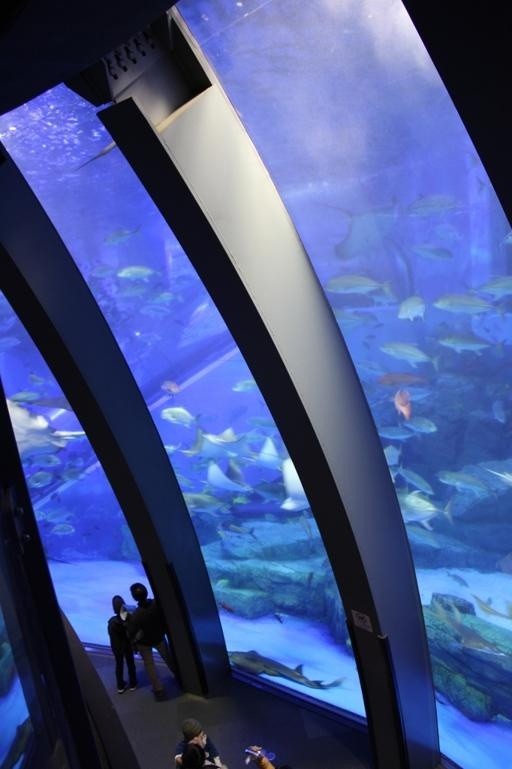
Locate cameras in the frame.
[244,747,264,759]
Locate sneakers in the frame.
[129,681,140,691]
[118,682,128,693]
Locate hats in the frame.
[182,718,202,739]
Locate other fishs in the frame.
[322,190,512,660]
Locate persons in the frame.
[123,583,186,702]
[108,595,138,694]
[176,718,222,764]
[174,744,221,769]
[251,745,276,768]
